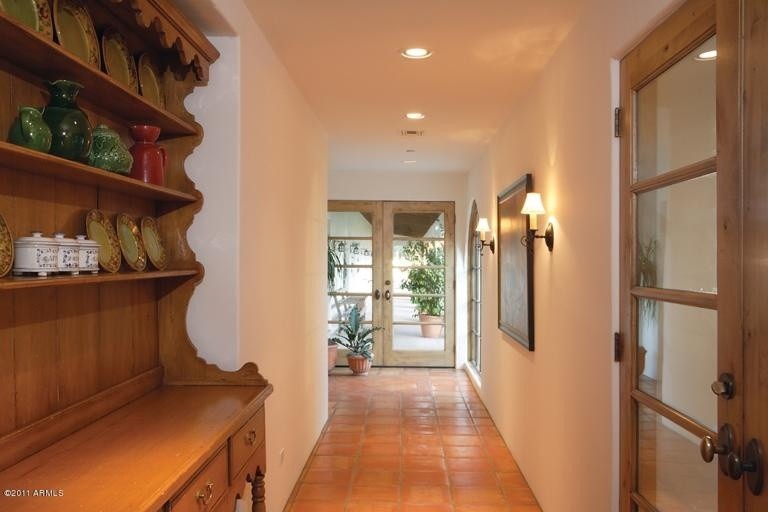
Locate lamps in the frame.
[332,240,370,256]
[520,191,554,252]
[475,217,495,255]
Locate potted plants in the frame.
[327,244,343,376]
[332,303,386,375]
[401,238,446,339]
[635,235,659,378]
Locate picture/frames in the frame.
[496,173,535,351]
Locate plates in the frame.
[103,27,138,93]
[53,0,101,71]
[86,209,121,273]
[0,212,14,277]
[139,53,166,110]
[141,215,167,271]
[0,0,53,40]
[116,213,148,272]
